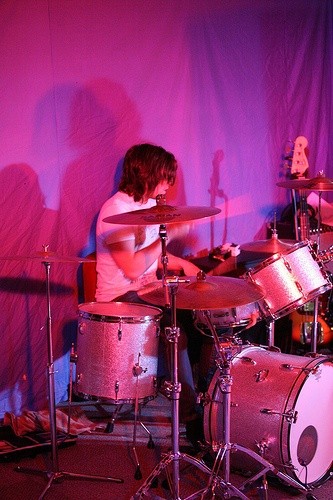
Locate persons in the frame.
[95,144,243,472]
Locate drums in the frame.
[74,301,163,404]
[200,345,333,491]
[237,237,333,323]
[192,298,264,328]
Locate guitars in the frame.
[284,135,333,346]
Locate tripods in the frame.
[11,261,321,500]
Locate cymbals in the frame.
[238,238,300,253]
[0,252,99,263]
[137,275,266,311]
[275,176,333,191]
[102,204,222,225]
